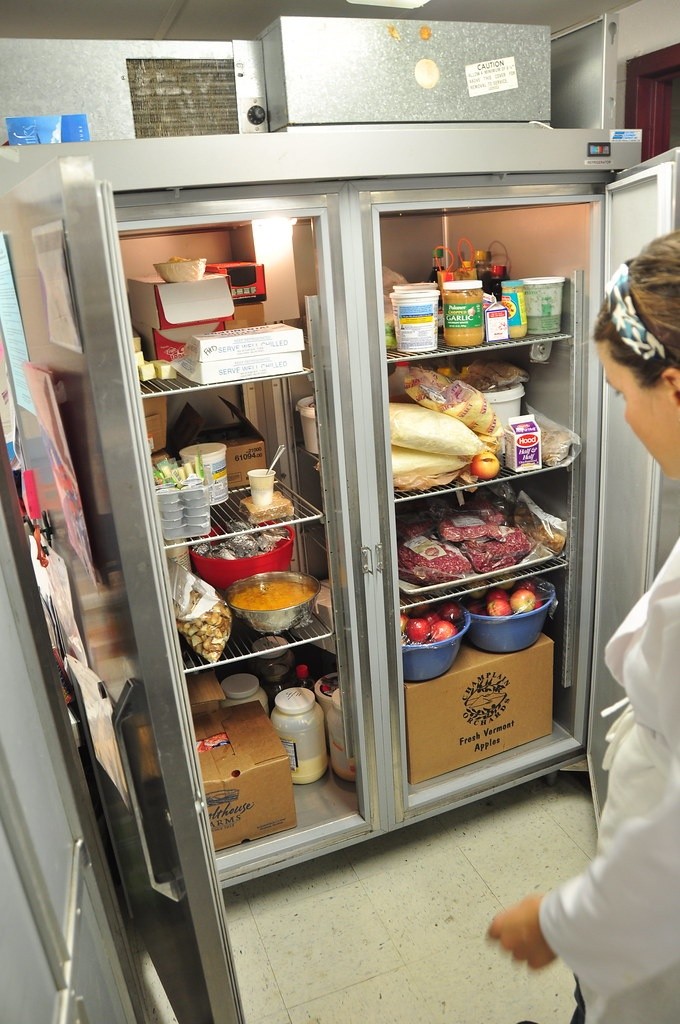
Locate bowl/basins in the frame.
[189,519,296,594]
[400,597,472,683]
[463,573,557,652]
[220,569,321,634]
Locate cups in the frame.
[246,469,276,508]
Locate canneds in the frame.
[442,279,485,347]
[501,280,527,338]
[221,636,357,783]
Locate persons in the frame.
[488,232,680,1024]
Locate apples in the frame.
[399,594,459,642]
[470,452,500,478]
[467,575,543,617]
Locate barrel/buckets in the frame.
[519,276,567,334]
[480,382,526,456]
[388,289,438,354]
[179,443,229,505]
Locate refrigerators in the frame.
[0,120,679,1024]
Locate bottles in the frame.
[220,636,360,788]
[499,279,529,339]
[428,246,510,347]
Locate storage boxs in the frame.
[140,380,167,453]
[124,699,296,864]
[207,261,266,296]
[402,632,553,787]
[126,275,234,331]
[221,298,264,331]
[129,316,225,362]
[169,395,267,489]
